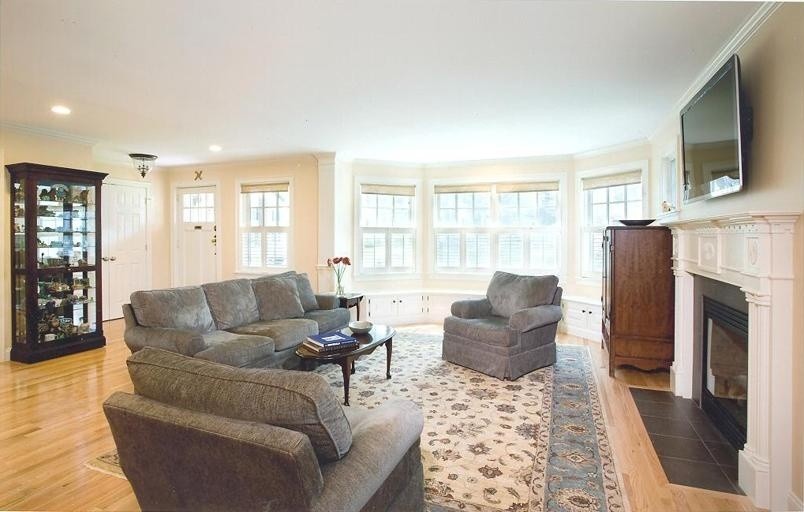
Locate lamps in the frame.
[129,154,158,178]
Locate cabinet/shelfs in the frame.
[5,162,109,364]
[601,227,674,378]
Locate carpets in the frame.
[83,329,628,512]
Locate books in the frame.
[300,331,359,354]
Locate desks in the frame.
[320,291,363,322]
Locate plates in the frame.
[619,219,655,226]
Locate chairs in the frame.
[102,345,424,512]
[441,271,563,380]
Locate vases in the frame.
[335,285,345,296]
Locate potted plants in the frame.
[327,257,351,296]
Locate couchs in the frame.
[122,271,351,372]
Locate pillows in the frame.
[253,275,305,321]
[284,272,320,312]
[130,287,217,332]
[126,346,353,462]
[486,271,559,320]
[202,279,261,329]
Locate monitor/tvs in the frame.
[679,53,753,204]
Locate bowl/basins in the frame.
[349,321,373,334]
